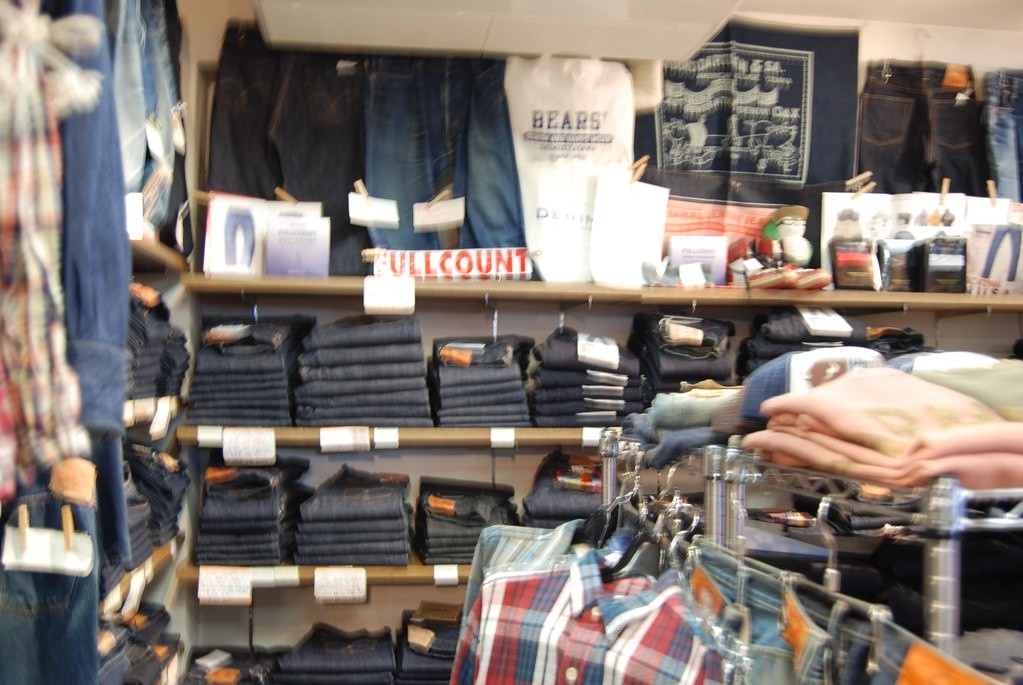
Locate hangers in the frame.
[478,442,885,672]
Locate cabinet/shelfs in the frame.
[176,272,1023,685]
[97,227,192,685]
[600,427,1023,657]
